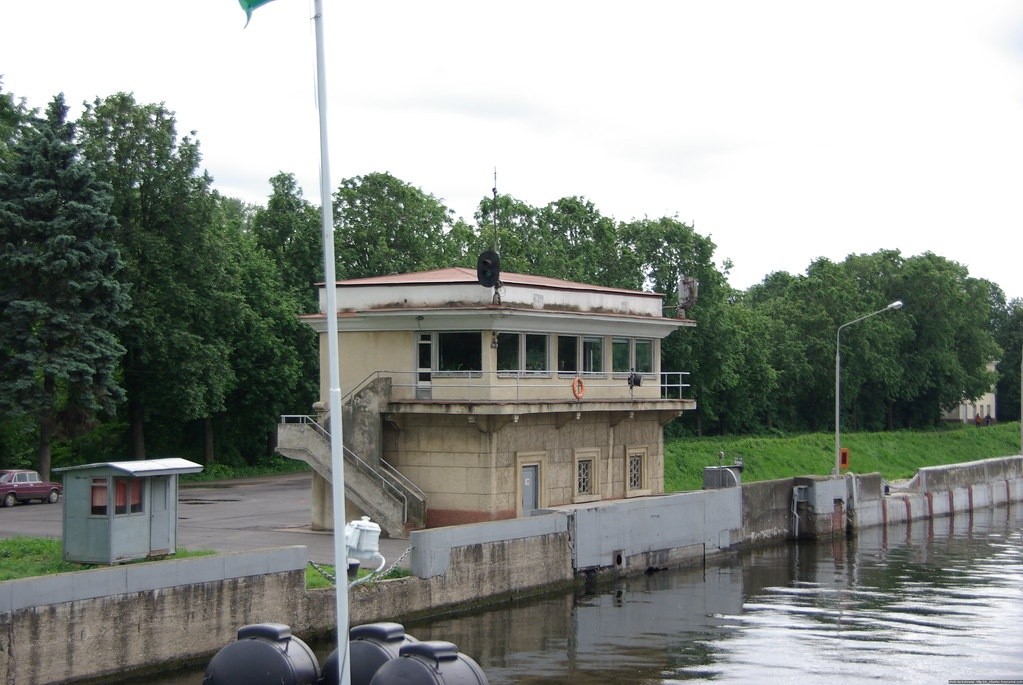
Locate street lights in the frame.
[834,301,903,475]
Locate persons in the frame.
[985,413,993,426]
[975,413,981,428]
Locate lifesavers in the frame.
[572,376,585,398]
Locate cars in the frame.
[0,468,63,507]
